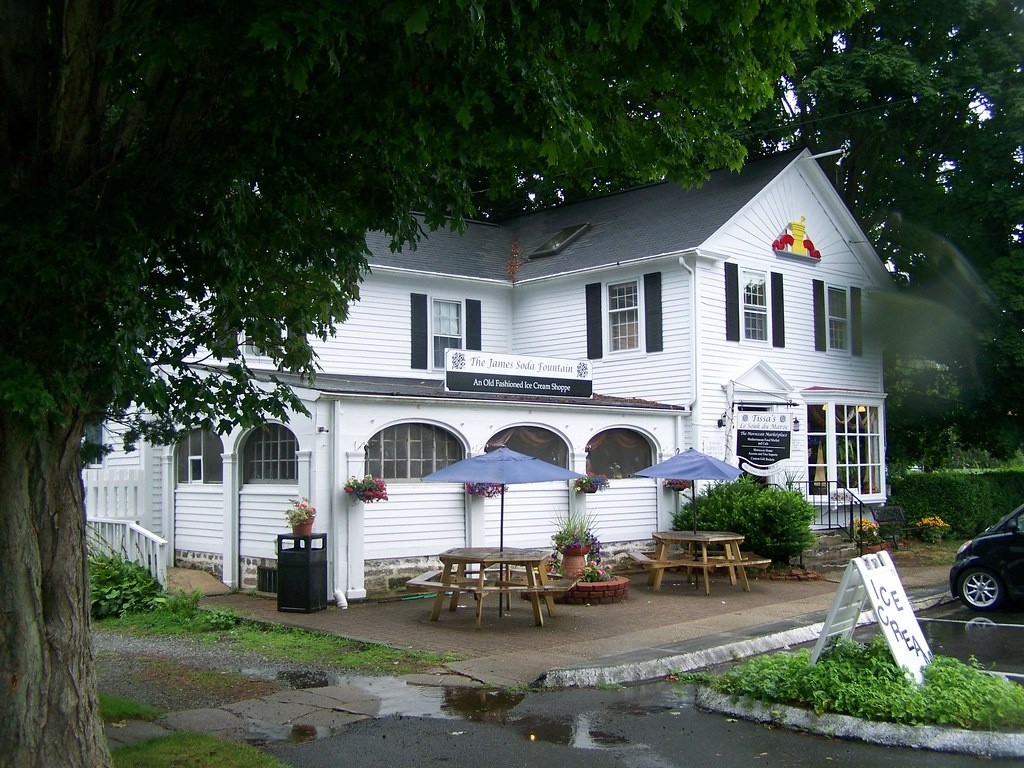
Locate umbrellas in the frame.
[633,447,745,589]
[420,445,586,617]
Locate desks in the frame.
[651,528,751,597]
[432,546,557,629]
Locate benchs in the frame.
[868,504,929,551]
[404,567,582,595]
[628,549,773,573]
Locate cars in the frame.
[949,504,1024,612]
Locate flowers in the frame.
[916,515,950,543]
[533,502,621,583]
[848,516,882,543]
[572,471,611,497]
[282,493,317,526]
[465,480,510,499]
[665,478,692,492]
[342,473,389,504]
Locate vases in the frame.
[583,484,599,494]
[858,539,868,547]
[559,543,591,575]
[673,486,685,492]
[292,514,316,535]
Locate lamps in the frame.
[792,417,799,432]
[859,405,866,412]
[822,405,826,411]
[717,411,727,427]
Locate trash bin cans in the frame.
[277,532,327,613]
